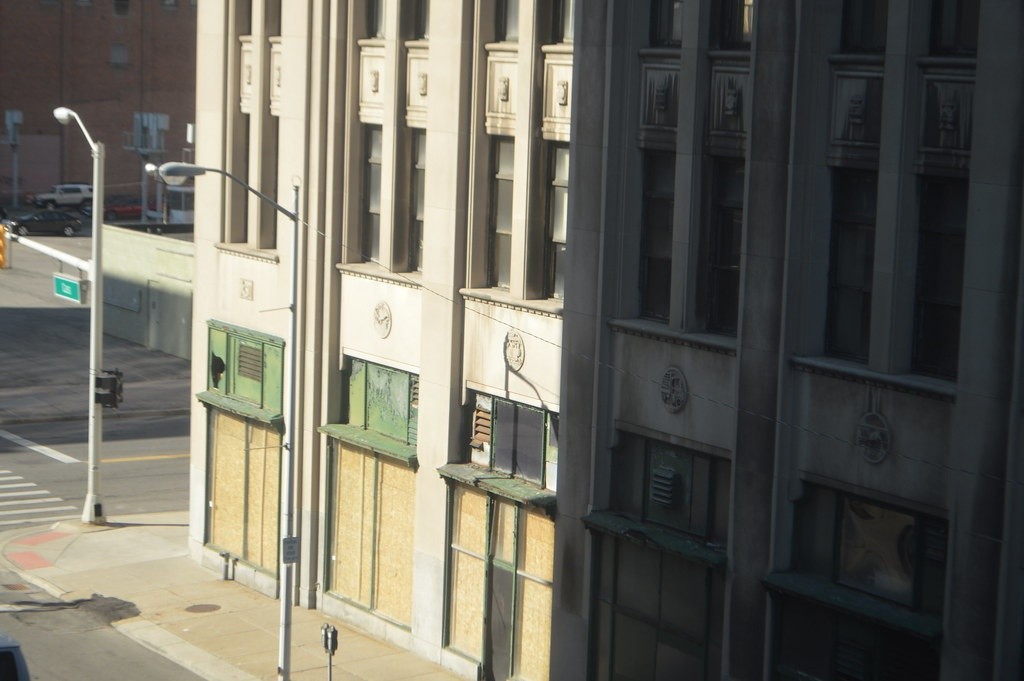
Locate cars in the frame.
[3,208,83,238]
[104,194,159,222]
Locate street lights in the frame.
[161,161,300,681]
[54,106,111,525]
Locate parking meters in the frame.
[321,623,338,681]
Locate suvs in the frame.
[33,183,94,214]
